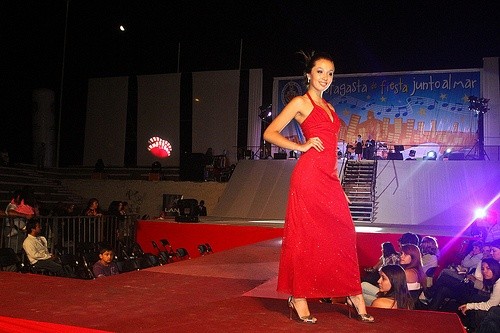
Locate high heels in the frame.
[346,295,375,321]
[287,295,317,324]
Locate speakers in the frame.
[431,269,471,299]
[175,198,199,222]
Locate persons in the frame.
[5,187,38,254]
[56,201,65,209]
[108,201,130,244]
[361,232,500,333]
[84,198,104,237]
[197,200,207,216]
[22,220,62,276]
[264,53,374,324]
[363,134,376,159]
[93,245,116,278]
[355,135,364,161]
